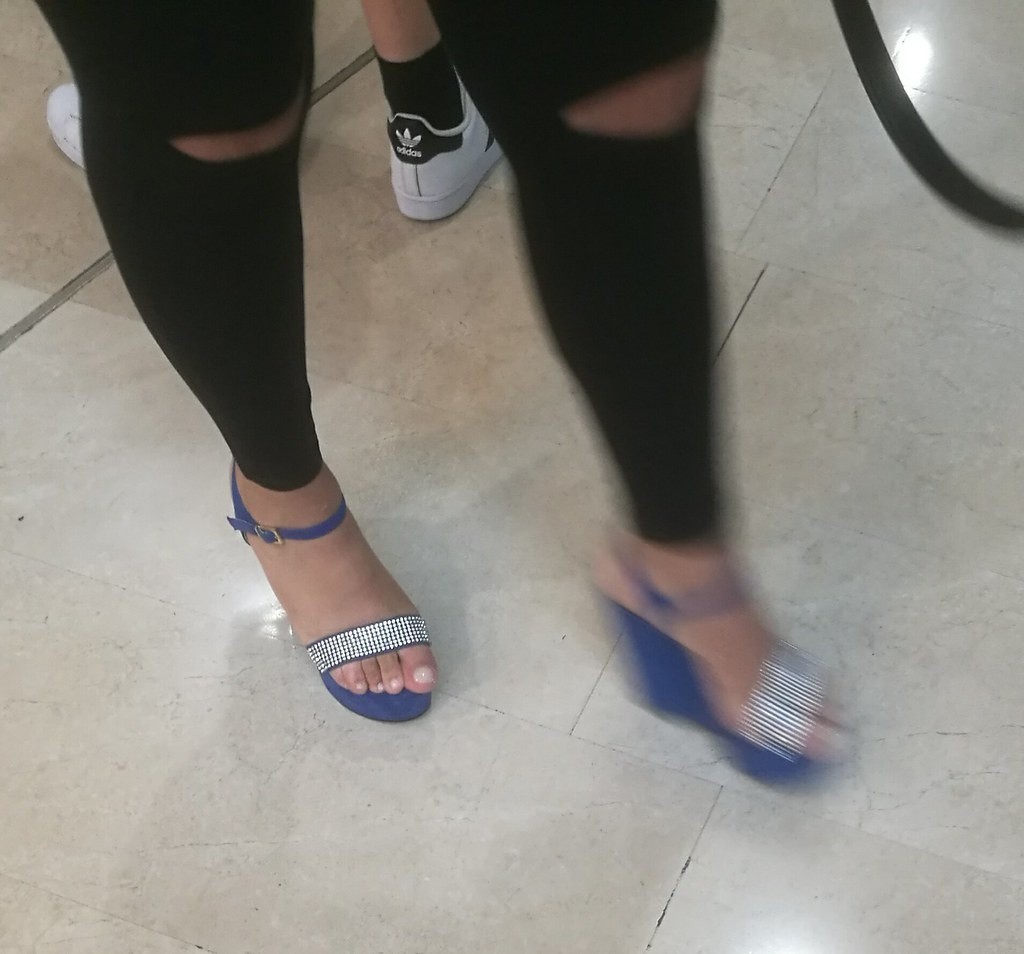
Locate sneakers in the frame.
[47,83,85,170]
[385,71,505,221]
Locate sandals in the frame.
[227,456,437,722]
[594,529,855,785]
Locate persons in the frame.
[38,0,861,785]
[45,0,504,220]
